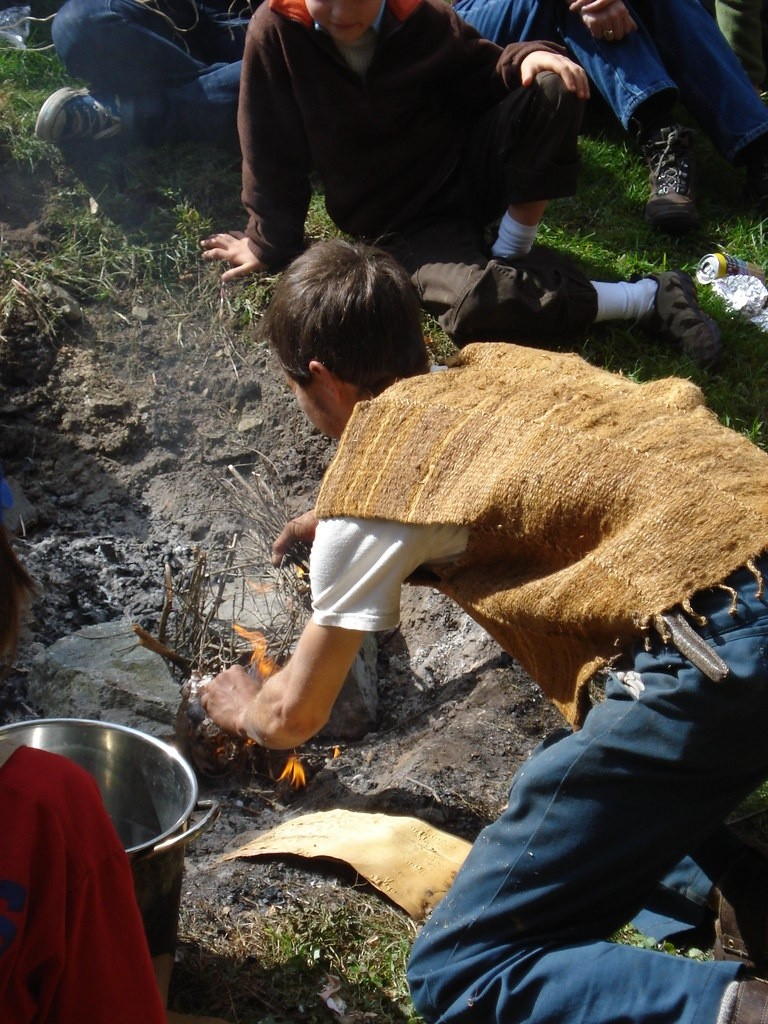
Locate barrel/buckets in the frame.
[0,717,220,969]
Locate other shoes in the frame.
[0,7,31,49]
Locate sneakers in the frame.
[747,154,768,206]
[33,81,130,152]
[644,126,702,233]
[646,268,724,374]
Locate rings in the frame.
[603,31,612,36]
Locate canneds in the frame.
[695,252,766,286]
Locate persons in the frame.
[204,241,768,1024]
[200,0,722,363]
[35,0,264,144]
[0,529,169,1024]
[451,0,768,227]
